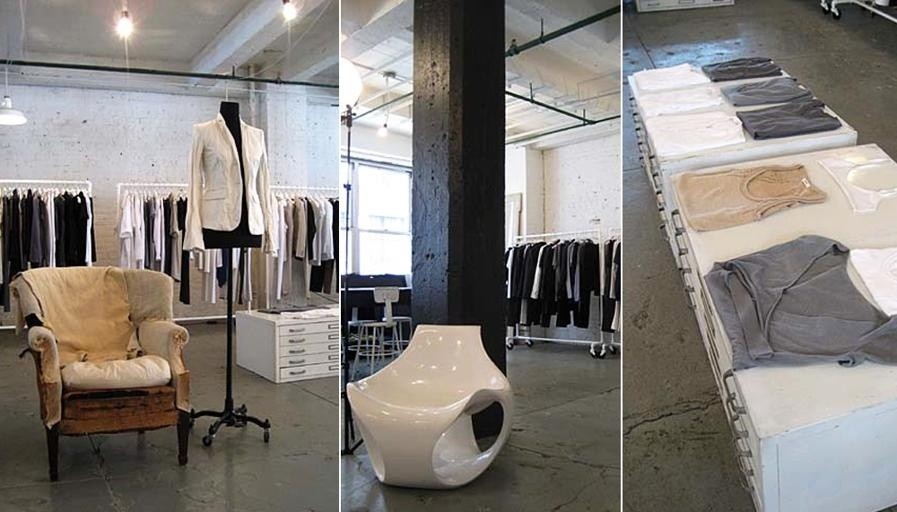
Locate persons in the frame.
[179,99,279,259]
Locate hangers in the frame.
[0,180,87,197]
[273,186,337,198]
[122,183,188,194]
[516,230,598,243]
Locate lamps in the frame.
[281,0,297,21]
[377,77,392,138]
[0,34,27,126]
[115,11,134,37]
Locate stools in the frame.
[382,317,412,359]
[348,320,384,366]
[350,321,401,382]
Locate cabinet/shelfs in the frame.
[665,144,897,512]
[234,310,339,383]
[627,61,857,219]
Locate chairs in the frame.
[346,324,513,489]
[10,267,190,482]
